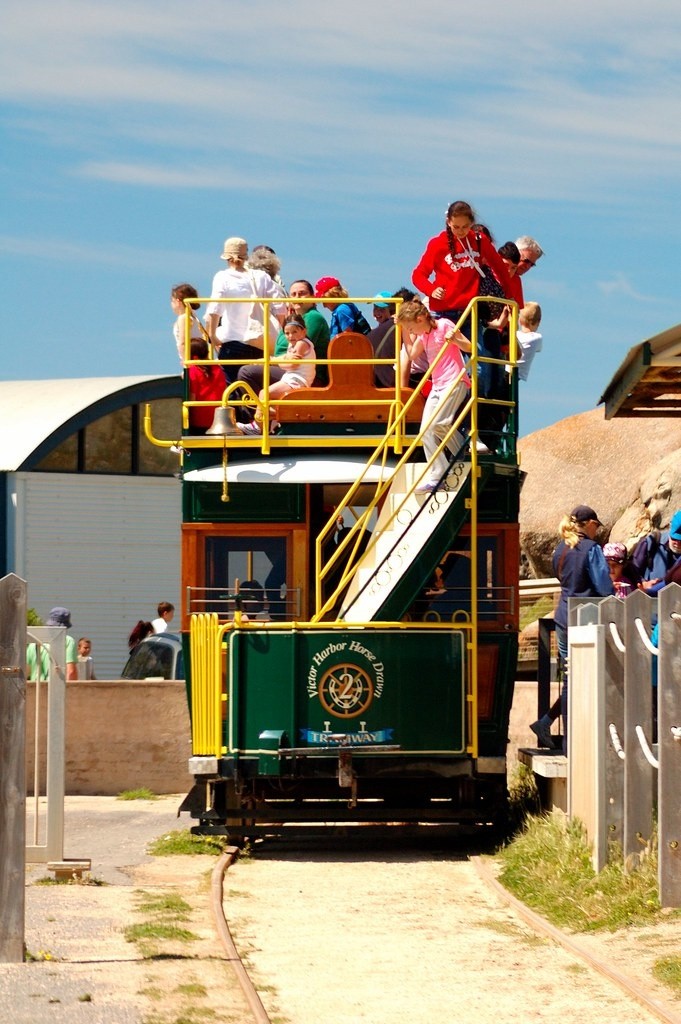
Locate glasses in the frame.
[593,520,599,528]
[520,257,536,268]
[605,556,623,564]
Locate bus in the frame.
[143,295,528,854]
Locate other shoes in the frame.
[268,420,282,434]
[414,480,446,494]
[467,431,488,452]
[529,720,554,747]
[236,420,261,435]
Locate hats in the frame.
[669,510,681,540]
[602,542,627,558]
[314,276,339,298]
[366,291,393,307]
[46,607,72,629]
[220,237,249,263]
[570,505,604,527]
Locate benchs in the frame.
[275,331,424,423]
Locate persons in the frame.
[127,620,155,650]
[26,607,78,681]
[171,200,545,454]
[328,511,494,619]
[152,602,176,634]
[76,638,96,680]
[528,505,681,758]
[262,536,287,619]
[392,302,468,494]
[233,580,265,621]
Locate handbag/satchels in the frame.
[242,271,282,355]
[475,230,506,327]
